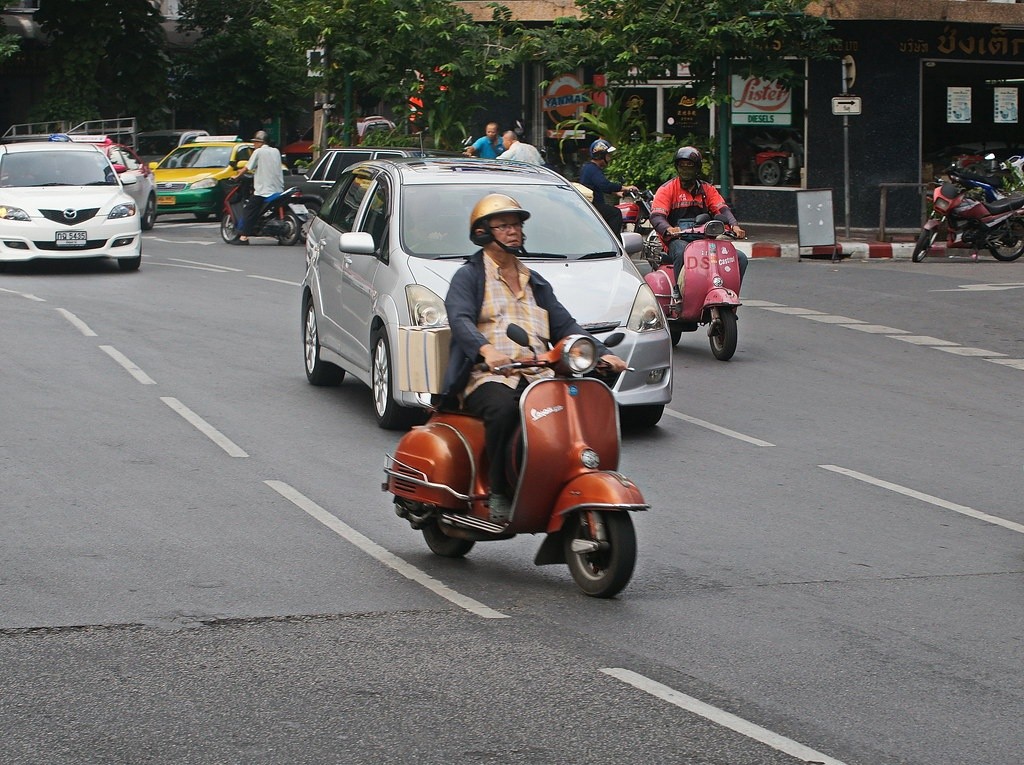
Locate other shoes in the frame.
[488,492,511,522]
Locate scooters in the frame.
[383,323,651,595]
[641,214,748,361]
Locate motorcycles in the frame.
[913,164,1024,265]
[615,188,654,257]
[222,161,305,248]
[984,151,1024,184]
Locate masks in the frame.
[677,166,696,179]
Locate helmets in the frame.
[251,131,268,144]
[590,139,616,161]
[468,192,532,238]
[674,146,702,174]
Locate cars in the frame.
[93,143,158,230]
[128,129,209,171]
[333,115,395,148]
[286,147,470,244]
[933,142,1024,172]
[300,158,672,428]
[0,142,142,272]
[149,143,288,223]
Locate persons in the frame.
[495,131,545,166]
[438,192,628,524]
[462,121,507,159]
[648,146,748,315]
[232,130,283,246]
[581,139,639,242]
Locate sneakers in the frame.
[231,238,249,246]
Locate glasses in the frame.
[490,222,521,229]
